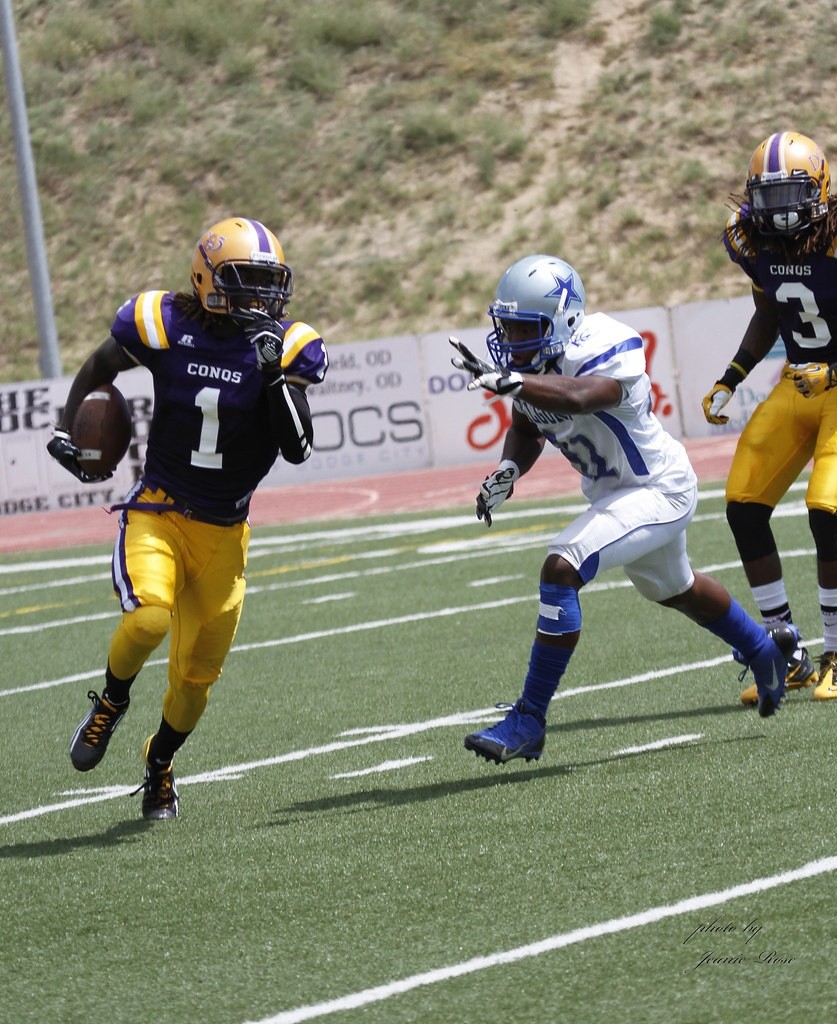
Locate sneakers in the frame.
[741,648,820,707]
[463,697,547,765]
[130,735,179,820]
[68,687,131,772]
[810,651,837,700]
[732,621,802,718]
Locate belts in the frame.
[103,477,249,526]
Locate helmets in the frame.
[746,132,831,239]
[192,217,293,325]
[486,255,585,373]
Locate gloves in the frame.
[788,362,837,399]
[449,337,523,401]
[477,460,520,527]
[46,428,116,484]
[702,381,737,424]
[243,308,286,377]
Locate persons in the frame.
[702,131,837,703]
[448,255,798,766]
[46,218,329,820]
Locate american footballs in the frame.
[70,382,134,479]
